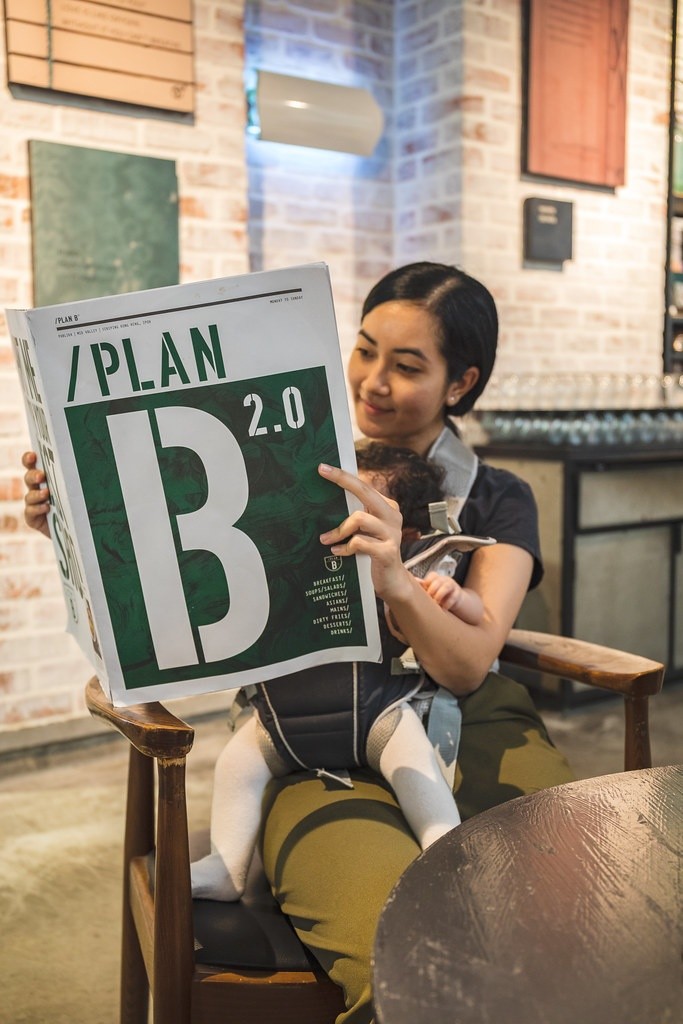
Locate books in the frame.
[2,262,383,711]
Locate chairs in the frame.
[76,603,670,1024]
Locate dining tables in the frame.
[364,759,682,1024]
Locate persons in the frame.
[16,262,548,1024]
[180,444,485,904]
